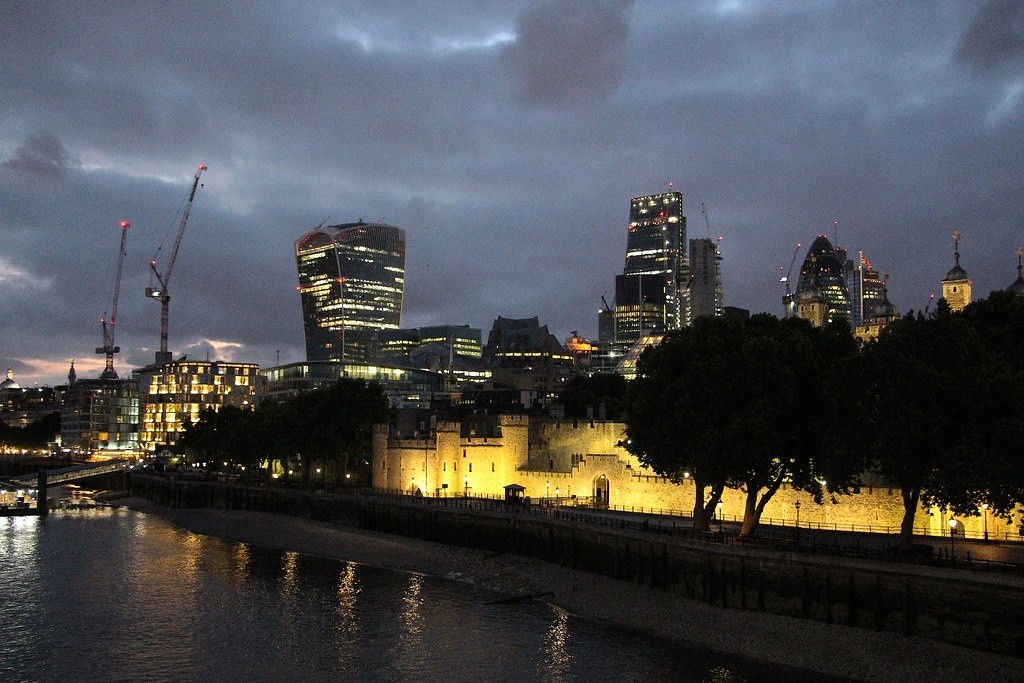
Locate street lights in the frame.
[316,466,321,488]
[949,516,958,568]
[464,476,469,497]
[545,481,550,508]
[411,478,415,496]
[717,500,724,539]
[981,502,990,544]
[344,473,350,495]
[555,486,560,511]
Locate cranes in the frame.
[699,201,723,255]
[778,243,801,296]
[96,220,133,382]
[145,164,208,365]
